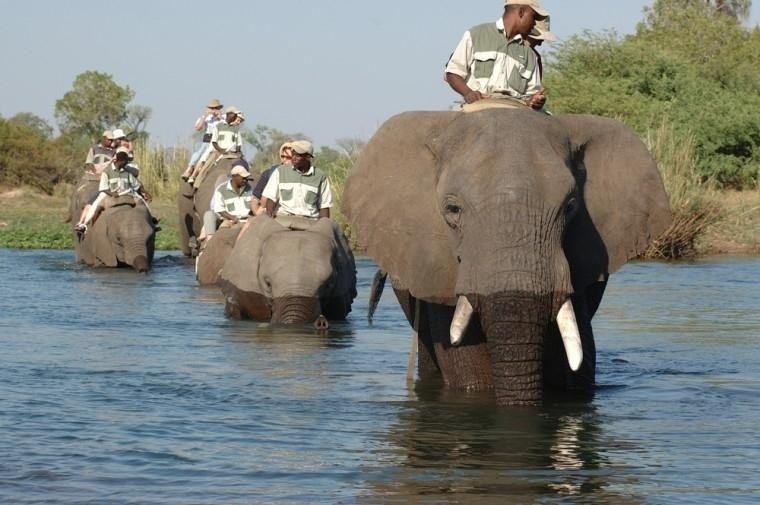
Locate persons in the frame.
[250,140,333,219]
[181,99,254,200]
[73,129,162,235]
[442,0,548,110]
[197,159,254,246]
[443,13,555,85]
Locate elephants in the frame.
[176,155,267,258]
[197,224,242,286]
[79,205,156,275]
[64,177,101,261]
[219,213,359,330]
[337,104,675,410]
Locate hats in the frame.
[208,98,223,109]
[232,158,250,173]
[527,13,560,43]
[278,142,292,157]
[103,130,133,158]
[288,139,315,157]
[503,1,549,21]
[116,151,133,162]
[231,165,251,178]
[226,106,246,121]
